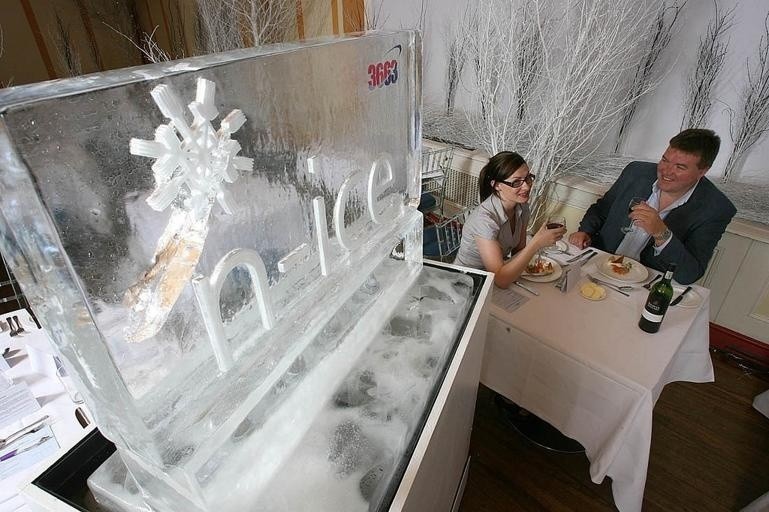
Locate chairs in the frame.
[419,144,461,226]
[431,207,468,266]
[0,253,32,315]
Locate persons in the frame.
[568,129,737,285]
[451,151,567,289]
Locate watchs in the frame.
[653,228,671,242]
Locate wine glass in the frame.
[544,215,567,252]
[620,197,648,237]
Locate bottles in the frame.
[637,261,676,335]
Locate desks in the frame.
[15,252,497,512]
[0,308,98,512]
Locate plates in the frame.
[515,255,562,283]
[672,284,702,308]
[576,282,607,301]
[594,254,649,284]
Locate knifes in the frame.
[669,286,693,306]
[512,279,539,297]
[566,249,598,268]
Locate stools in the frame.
[419,223,458,261]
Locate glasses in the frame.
[498,173,537,189]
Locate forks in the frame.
[586,273,633,297]
[643,274,662,289]
